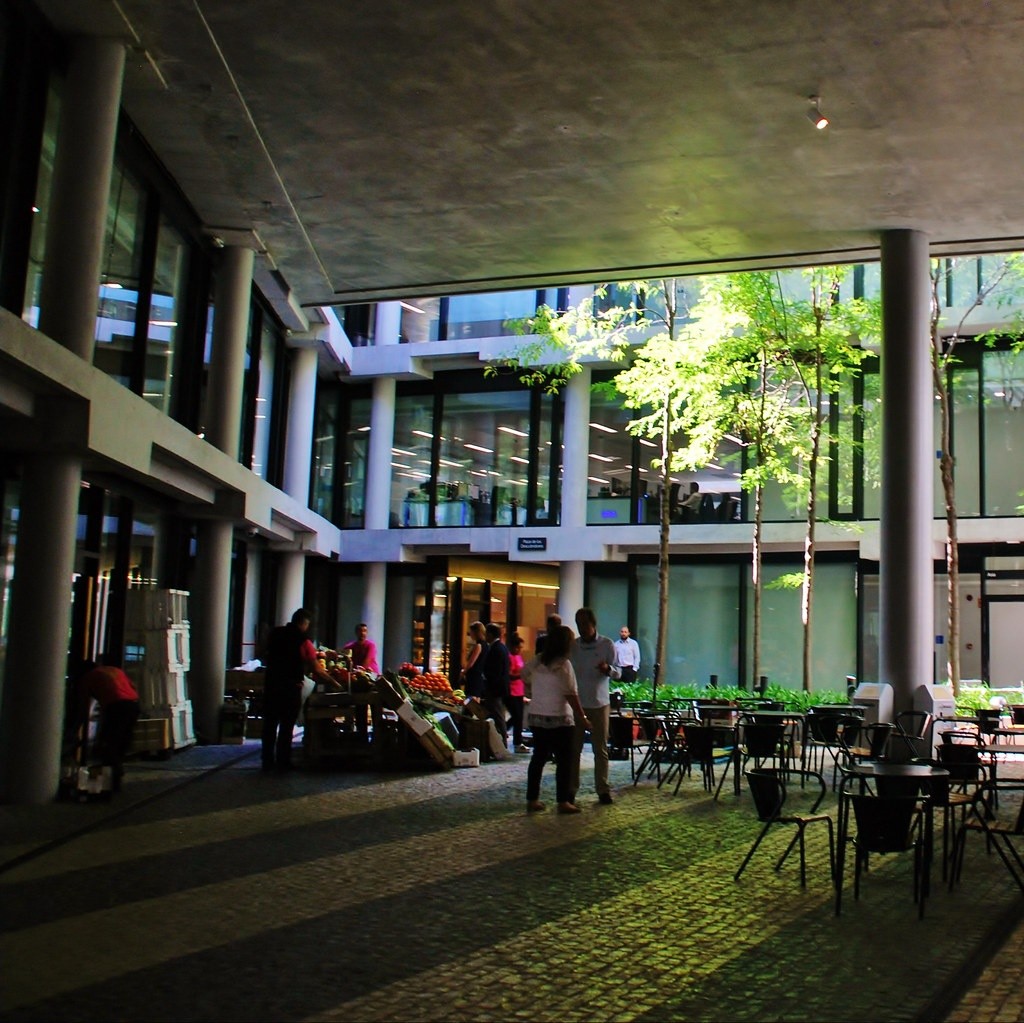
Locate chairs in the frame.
[669,483,681,514]
[607,689,1024,922]
[700,494,714,523]
[722,500,738,521]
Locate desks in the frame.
[404,500,527,527]
[587,496,683,524]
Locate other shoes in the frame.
[276,753,297,773]
[527,800,547,812]
[256,759,275,775]
[556,802,582,813]
[598,792,612,804]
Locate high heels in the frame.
[514,742,530,753]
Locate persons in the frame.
[338,623,384,733]
[80,660,142,792]
[460,620,490,704]
[636,628,655,683]
[568,607,623,805]
[524,625,594,814]
[535,613,562,656]
[260,608,346,772]
[678,481,703,516]
[505,631,530,753]
[485,622,510,749]
[612,626,641,686]
